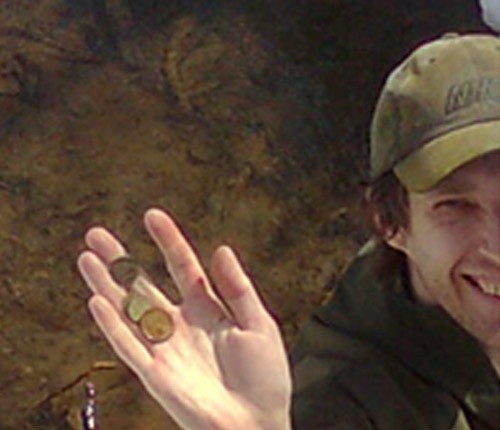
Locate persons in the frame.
[76,28,500,430]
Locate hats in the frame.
[371,31,500,191]
